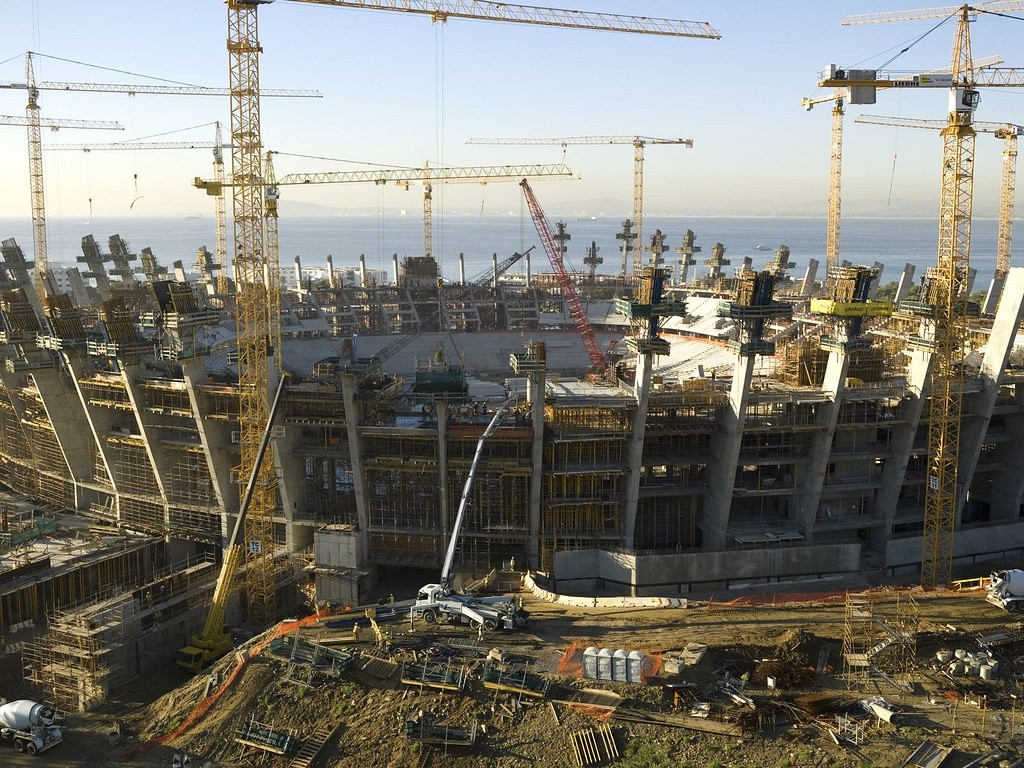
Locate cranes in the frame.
[801,0,1024,590]
[465,136,694,300]
[193,0,720,638]
[0,51,573,381]
[519,177,620,376]
[366,244,536,364]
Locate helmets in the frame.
[512,557,514,558]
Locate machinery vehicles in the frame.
[176,373,289,675]
[415,396,530,635]
[0,698,63,756]
[985,569,1024,611]
